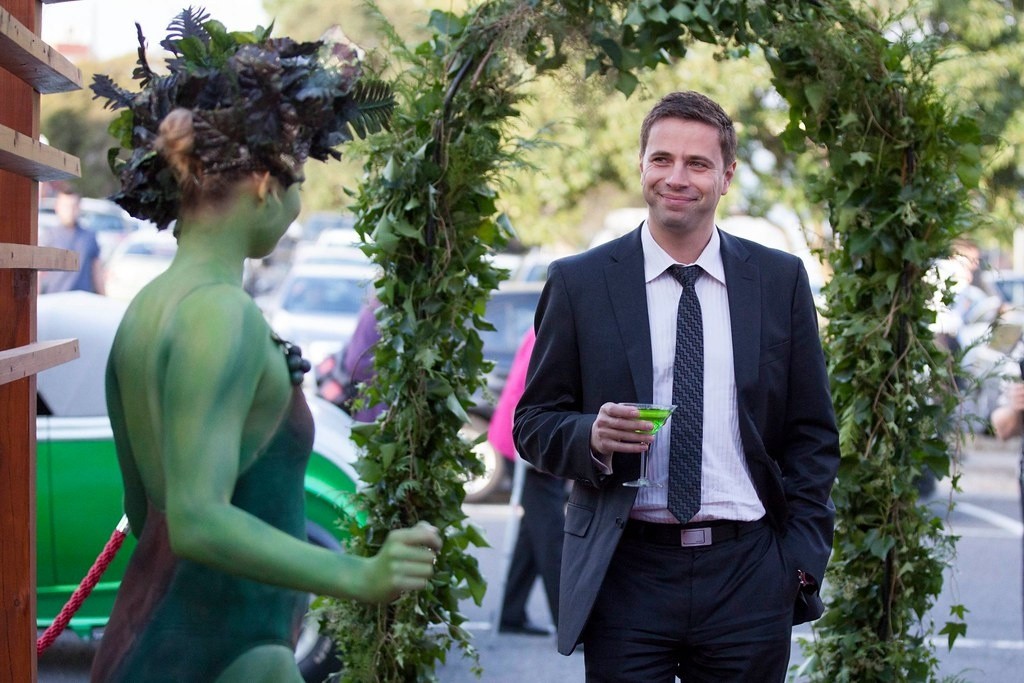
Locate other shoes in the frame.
[497,623,549,637]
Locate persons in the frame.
[499,464,565,639]
[991,354,1024,527]
[345,295,395,423]
[511,92,842,683]
[86,5,442,683]
[38,189,104,297]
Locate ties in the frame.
[665,265,705,525]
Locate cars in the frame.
[35,201,1023,655]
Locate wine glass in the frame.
[617,402,678,488]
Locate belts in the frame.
[626,518,764,548]
